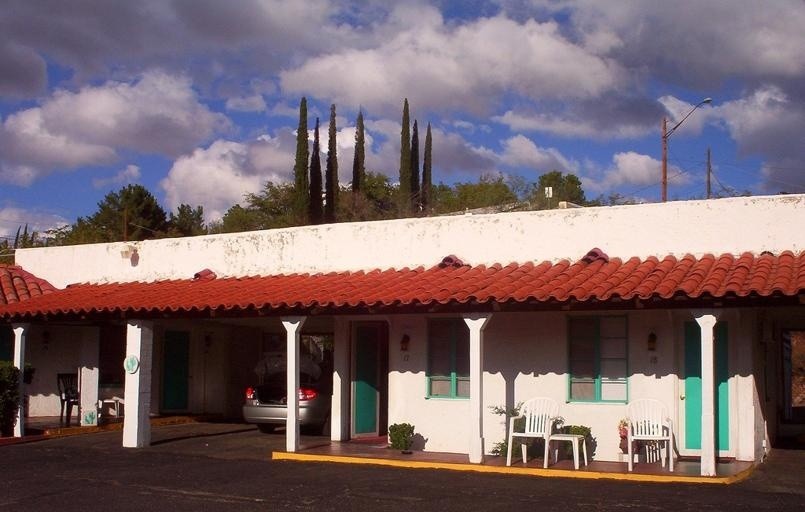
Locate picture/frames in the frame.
[123,355,138,374]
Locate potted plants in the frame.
[389,423,415,454]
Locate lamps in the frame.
[649,333,656,350]
[401,336,410,351]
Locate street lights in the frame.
[661,97,713,202]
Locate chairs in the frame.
[57,373,78,428]
[624,398,673,471]
[506,397,560,468]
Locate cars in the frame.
[242,350,334,436]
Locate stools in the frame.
[549,434,587,470]
